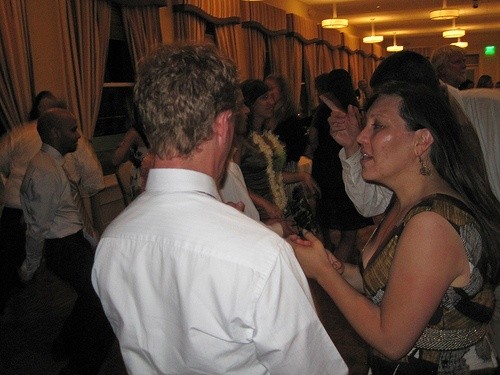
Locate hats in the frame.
[243,79,274,105]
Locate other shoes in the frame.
[60,367,81,375]
[50,337,71,361]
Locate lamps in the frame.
[451,37,469,48]
[386,33,403,53]
[363,19,383,43]
[442,18,465,38]
[321,1,348,28]
[428,0,459,20]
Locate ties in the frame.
[62,160,93,238]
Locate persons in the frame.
[0,41,500,374]
